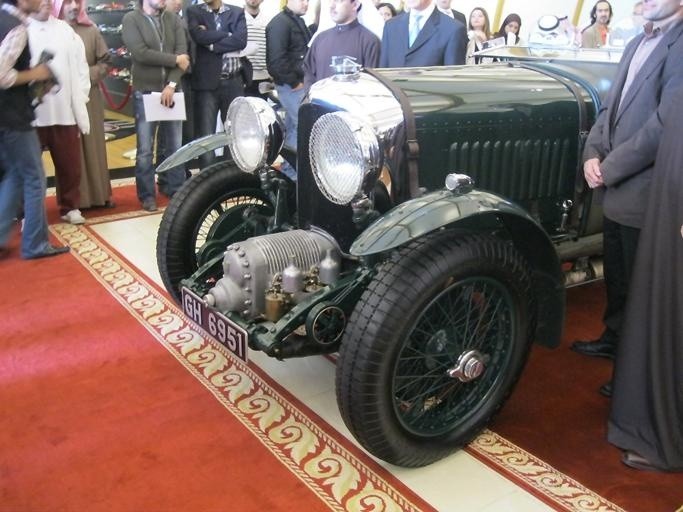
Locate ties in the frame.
[408,16,422,48]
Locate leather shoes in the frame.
[142,197,157,211]
[624,452,656,470]
[573,338,616,358]
[35,245,69,256]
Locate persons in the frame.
[571,0,683,397]
[239,1,271,100]
[265,0,321,185]
[166,1,195,180]
[187,0,248,171]
[1,1,114,260]
[580,0,613,49]
[121,0,190,211]
[301,1,382,93]
[469,8,523,65]
[377,1,468,67]
[606,81,683,473]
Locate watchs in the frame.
[167,82,177,88]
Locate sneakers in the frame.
[61,209,85,224]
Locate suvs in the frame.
[147,13,632,472]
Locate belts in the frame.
[220,72,242,79]
